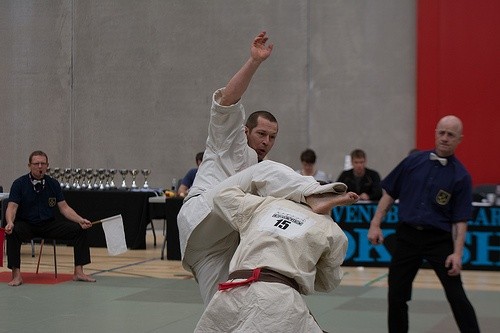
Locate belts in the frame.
[229,268,301,294]
[184,193,200,203]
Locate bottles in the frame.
[171,178,178,196]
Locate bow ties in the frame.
[429,151,447,166]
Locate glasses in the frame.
[31,162,49,168]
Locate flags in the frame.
[102,214,127,256]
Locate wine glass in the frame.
[129,169,139,188]
[109,169,117,189]
[44,167,110,190]
[141,169,151,188]
[119,169,128,188]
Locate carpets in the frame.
[0,271,74,285]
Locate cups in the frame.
[487,192,496,203]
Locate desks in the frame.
[166,196,500,271]
[57,186,166,249]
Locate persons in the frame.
[366,115,480,333]
[5,150,96,286]
[193,186,347,333]
[295,149,329,183]
[337,149,382,202]
[177,152,204,194]
[176,31,361,309]
